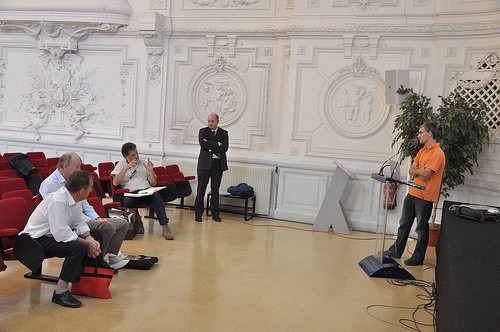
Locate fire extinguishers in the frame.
[383,181,397,210]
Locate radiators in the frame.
[161,156,272,215]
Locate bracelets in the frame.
[84,235,91,239]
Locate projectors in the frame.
[457,205,500,223]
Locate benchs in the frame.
[0,152,195,283]
[206,191,256,221]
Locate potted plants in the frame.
[391,84,490,246]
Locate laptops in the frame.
[389,160,407,182]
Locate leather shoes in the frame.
[52,290,82,308]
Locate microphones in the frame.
[378,150,403,180]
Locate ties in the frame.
[212,131,215,134]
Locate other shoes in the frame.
[212,215,221,222]
[404,258,423,266]
[162,226,174,240]
[137,220,144,234]
[195,216,202,222]
[378,250,400,259]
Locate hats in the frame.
[108,251,130,270]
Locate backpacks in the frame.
[8,153,37,177]
[227,183,255,197]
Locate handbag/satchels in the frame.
[27,174,44,195]
[68,256,114,299]
[122,212,137,240]
[123,255,159,270]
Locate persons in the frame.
[39,152,129,274]
[70,103,90,144]
[110,142,174,240]
[335,86,373,125]
[378,122,446,266]
[200,83,236,117]
[23,99,49,141]
[195,113,229,222]
[18,170,104,307]
